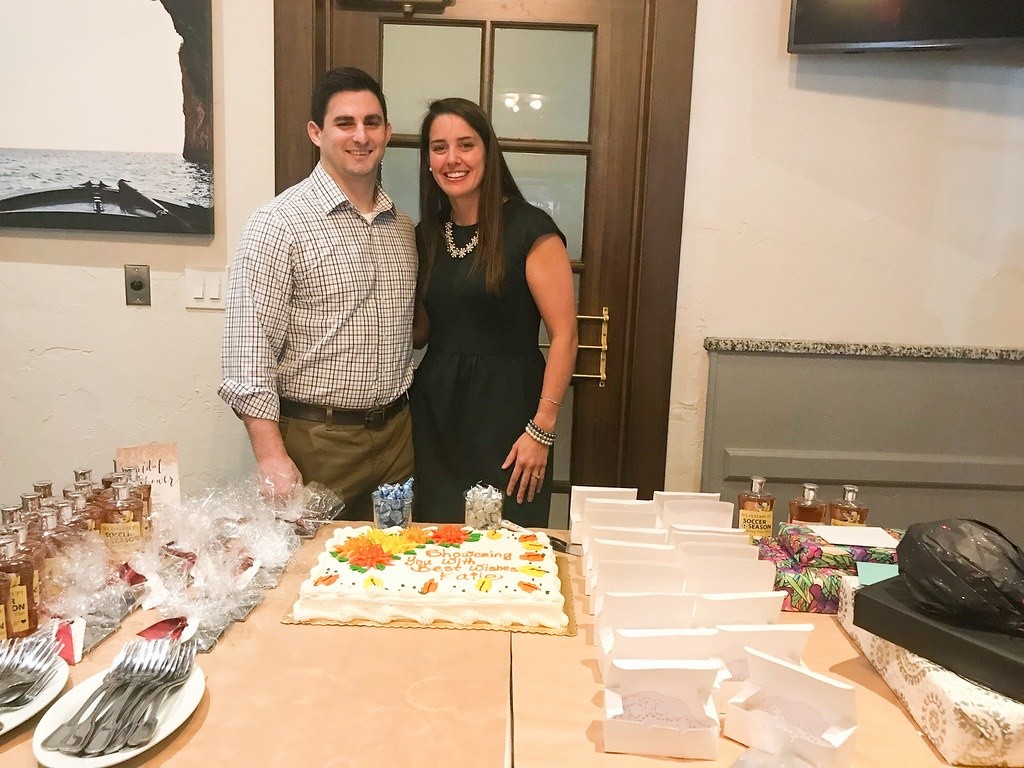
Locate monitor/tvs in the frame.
[787,0,1024,55]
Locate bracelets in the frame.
[539,396,562,408]
[525,419,557,447]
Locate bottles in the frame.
[0,468,153,642]
[738,477,776,543]
[788,482,828,525]
[830,484,869,527]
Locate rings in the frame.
[531,475,540,479]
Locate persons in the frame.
[219,64,414,526]
[413,97,578,535]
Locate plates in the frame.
[32,662,206,768]
[0,656,69,737]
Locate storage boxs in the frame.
[836,576,1024,768]
[371,490,412,529]
[752,535,857,614]
[570,486,638,544]
[584,497,815,676]
[462,488,504,530]
[777,521,907,568]
[853,575,1024,702]
[723,646,863,767]
[602,659,722,760]
[653,491,721,502]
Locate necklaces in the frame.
[444,207,481,260]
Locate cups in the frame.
[372,491,414,529]
[462,488,504,531]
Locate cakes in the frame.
[291,525,570,629]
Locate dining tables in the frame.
[0,520,952,767]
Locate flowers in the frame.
[361,527,389,544]
[429,523,481,549]
[397,524,434,544]
[381,533,425,555]
[329,533,374,563]
[347,543,401,573]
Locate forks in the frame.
[0,637,63,712]
[41,638,197,756]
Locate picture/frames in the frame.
[1,1,216,236]
